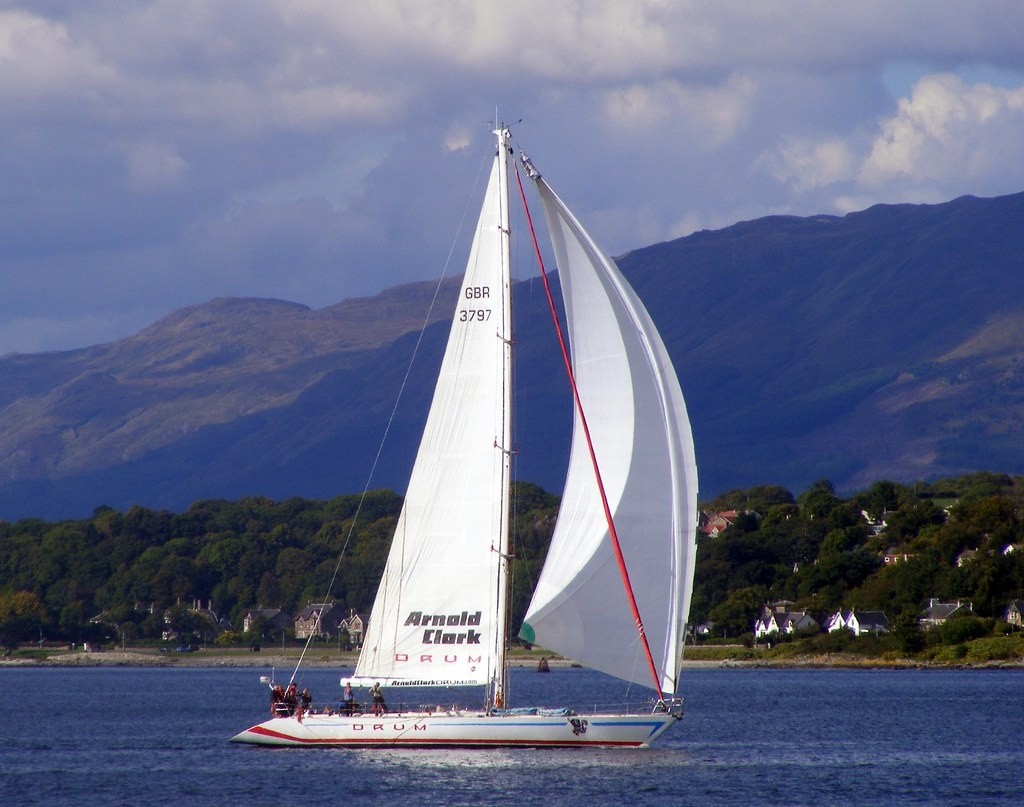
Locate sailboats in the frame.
[229,102,701,749]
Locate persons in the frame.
[344,682,353,717]
[269,683,311,716]
[369,682,388,716]
